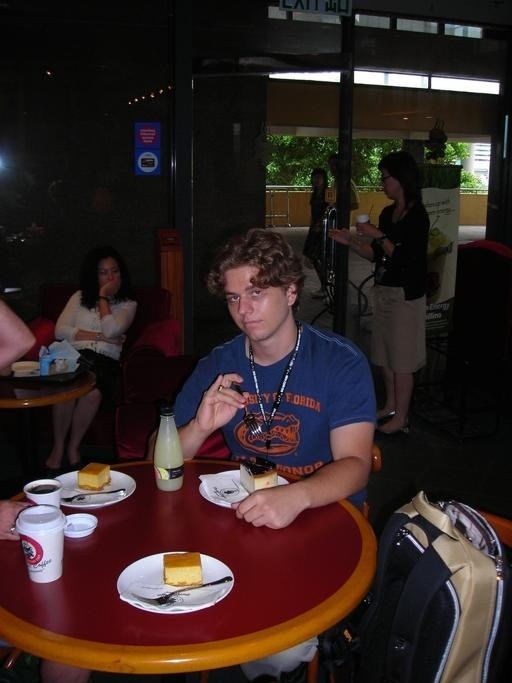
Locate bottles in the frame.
[153,407,186,490]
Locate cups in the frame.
[356,213,369,236]
[16,505,68,585]
[423,230,455,305]
[23,479,62,510]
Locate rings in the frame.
[10,526,14,535]
[219,384,223,391]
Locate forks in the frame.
[234,382,263,438]
[61,488,126,503]
[132,575,232,605]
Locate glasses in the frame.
[380,174,393,184]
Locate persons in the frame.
[327,150,428,438]
[0,282,37,371]
[43,245,136,470]
[327,157,360,225]
[304,170,337,297]
[0,485,92,683]
[144,229,376,531]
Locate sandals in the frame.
[375,408,396,421]
[377,422,410,437]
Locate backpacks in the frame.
[320,492,512,683]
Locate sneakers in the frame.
[311,288,329,296]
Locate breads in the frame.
[163,551,203,586]
[77,462,111,490]
[239,456,278,494]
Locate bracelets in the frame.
[376,236,387,241]
[96,332,100,342]
[101,295,110,300]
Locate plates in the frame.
[200,470,290,508]
[119,549,234,613]
[55,468,138,509]
[12,360,40,374]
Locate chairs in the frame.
[39,283,183,448]
[199,442,383,683]
[446,239,512,420]
